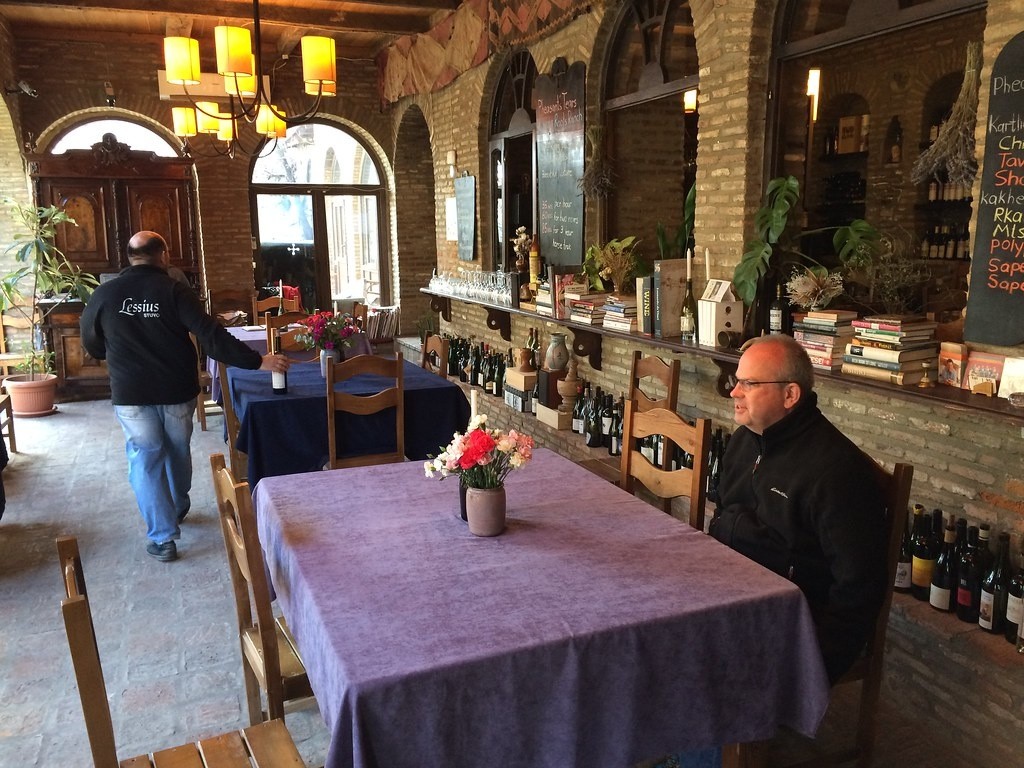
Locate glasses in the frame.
[728,374,802,393]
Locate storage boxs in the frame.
[534,402,573,430]
[504,367,538,413]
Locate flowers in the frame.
[424,414,535,489]
[582,237,644,289]
[294,307,361,351]
[509,226,531,258]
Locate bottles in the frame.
[271,330,287,394]
[928,115,947,142]
[531,371,539,415]
[526,327,539,369]
[892,134,900,163]
[823,172,865,200]
[929,170,973,200]
[572,381,732,502]
[536,260,544,281]
[825,126,838,155]
[920,220,970,259]
[431,332,513,397]
[278,297,288,331]
[529,234,539,256]
[541,263,548,285]
[770,282,787,335]
[893,503,1024,652]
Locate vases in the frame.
[612,286,624,297]
[514,253,532,301]
[319,349,339,379]
[466,482,506,537]
[811,304,823,312]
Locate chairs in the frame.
[56,537,307,768]
[252,297,368,362]
[621,398,712,534]
[420,330,450,378]
[210,453,314,728]
[0,387,16,452]
[719,457,913,768]
[217,359,249,483]
[574,350,682,516]
[189,329,225,432]
[0,295,46,393]
[322,352,409,472]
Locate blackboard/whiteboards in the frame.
[530,59,585,266]
[961,31,1024,349]
[456,175,475,260]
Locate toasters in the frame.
[681,278,697,340]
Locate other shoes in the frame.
[177,494,191,524]
[147,540,177,561]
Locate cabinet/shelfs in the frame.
[914,142,974,265]
[21,132,206,404]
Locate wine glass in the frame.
[429,269,512,307]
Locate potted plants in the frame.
[0,198,101,417]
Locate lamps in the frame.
[172,91,287,161]
[164,0,337,125]
[683,76,697,114]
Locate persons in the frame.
[80,229,290,562]
[941,358,959,386]
[524,235,531,262]
[970,363,999,380]
[702,335,886,705]
[514,226,526,261]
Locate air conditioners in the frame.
[158,70,271,106]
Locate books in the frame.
[598,294,637,334]
[242,324,266,331]
[367,304,402,339]
[535,288,551,317]
[791,308,859,371]
[549,266,590,320]
[841,314,942,385]
[563,291,613,327]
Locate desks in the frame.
[214,349,471,494]
[251,445,825,768]
[206,326,372,400]
